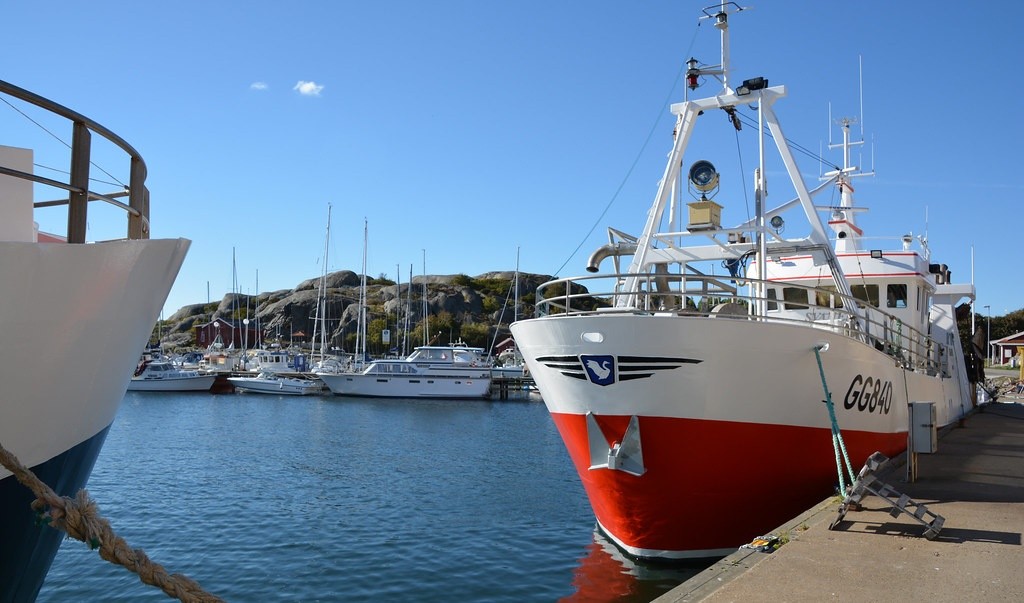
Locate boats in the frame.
[506,1,979,566]
[0,76,194,603]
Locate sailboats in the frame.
[126,199,535,400]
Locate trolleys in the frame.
[812,340,948,544]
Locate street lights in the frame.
[982,305,991,365]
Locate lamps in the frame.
[870,250,882,258]
